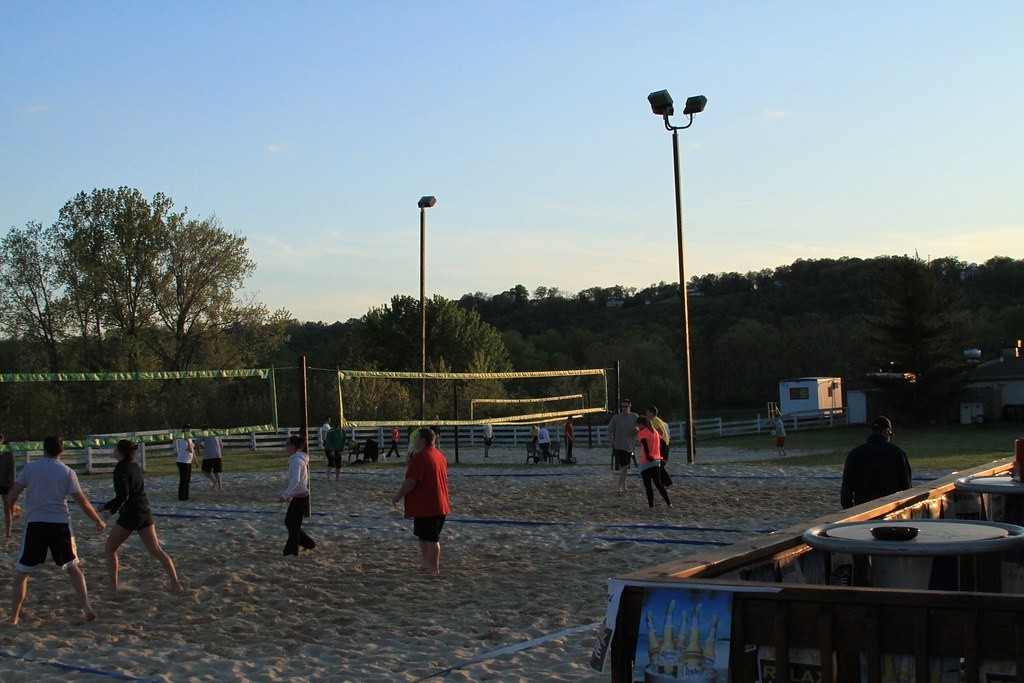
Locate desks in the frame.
[802,519,1024,589]
[955,476,1024,522]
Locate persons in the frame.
[201,424,225,490]
[483,416,496,458]
[564,416,575,463]
[537,423,551,463]
[391,426,451,573]
[95,439,185,594]
[773,413,787,456]
[840,416,912,509]
[0,433,15,538]
[324,418,346,481]
[647,405,674,488]
[685,415,696,463]
[176,424,200,501]
[322,417,331,447]
[429,415,442,451]
[1,435,106,625]
[636,415,674,509]
[530,425,540,444]
[279,436,319,557]
[387,426,401,457]
[607,399,640,491]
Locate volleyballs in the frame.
[771,430,776,436]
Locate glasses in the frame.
[888,428,895,439]
[622,406,630,408]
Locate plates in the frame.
[870,526,919,541]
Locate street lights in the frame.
[415,195,438,422]
[647,89,707,466]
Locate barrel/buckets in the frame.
[644,664,718,683]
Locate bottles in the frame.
[1015,437,1024,482]
[646,601,718,678]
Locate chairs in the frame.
[526,441,543,465]
[347,440,365,462]
[377,447,387,462]
[549,441,562,465]
